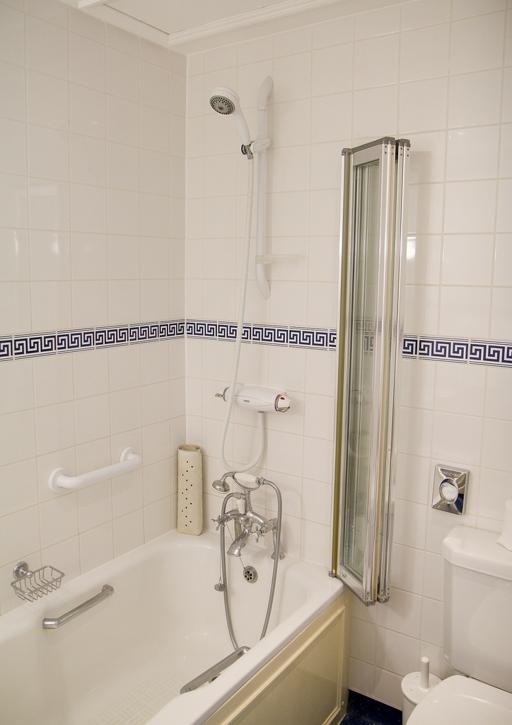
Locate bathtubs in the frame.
[0,531,349,725]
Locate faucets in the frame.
[227,530,250,556]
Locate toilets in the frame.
[407,525,512,725]
